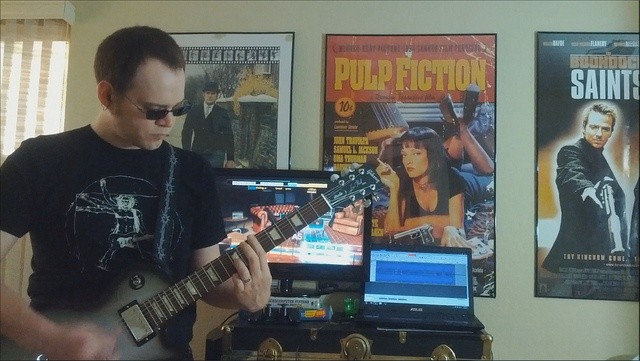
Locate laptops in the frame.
[354,243,486,332]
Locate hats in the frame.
[203,83,220,92]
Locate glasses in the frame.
[112,84,191,120]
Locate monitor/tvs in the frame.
[211,168,372,298]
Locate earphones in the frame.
[106,93,112,103]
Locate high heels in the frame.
[463,83,480,121]
[439,93,459,136]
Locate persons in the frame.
[0,26,272,361]
[375,84,493,236]
[181,82,235,169]
[542,104,627,274]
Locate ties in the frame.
[205,106,210,117]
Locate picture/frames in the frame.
[321,31,498,299]
[535,31,637,303]
[164,30,296,170]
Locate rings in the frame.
[240,277,253,284]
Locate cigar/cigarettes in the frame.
[377,158,390,167]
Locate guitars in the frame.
[0,158,382,361]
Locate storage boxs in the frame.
[219,319,493,361]
